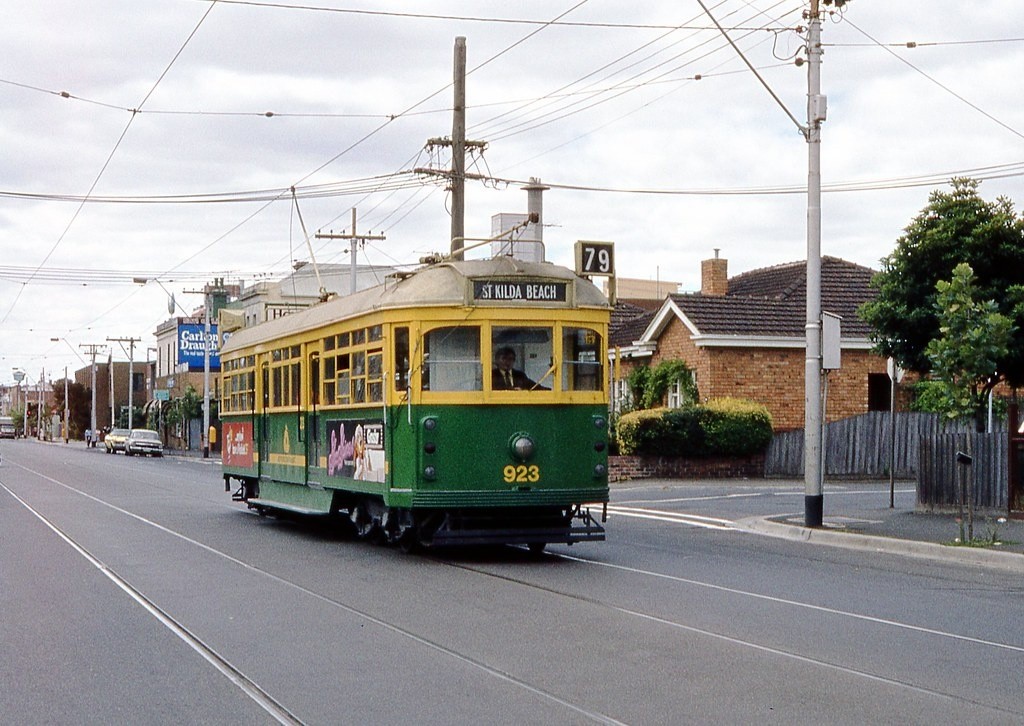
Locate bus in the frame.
[214,208,623,558]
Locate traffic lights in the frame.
[27,410,31,415]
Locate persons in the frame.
[103,424,117,435]
[85,427,91,447]
[492,347,552,390]
[96,427,101,446]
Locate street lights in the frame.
[12,366,42,440]
[50,337,97,448]
[132,277,211,459]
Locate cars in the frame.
[104,429,131,455]
[0,416,16,440]
[123,428,163,458]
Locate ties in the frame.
[505,371,512,390]
